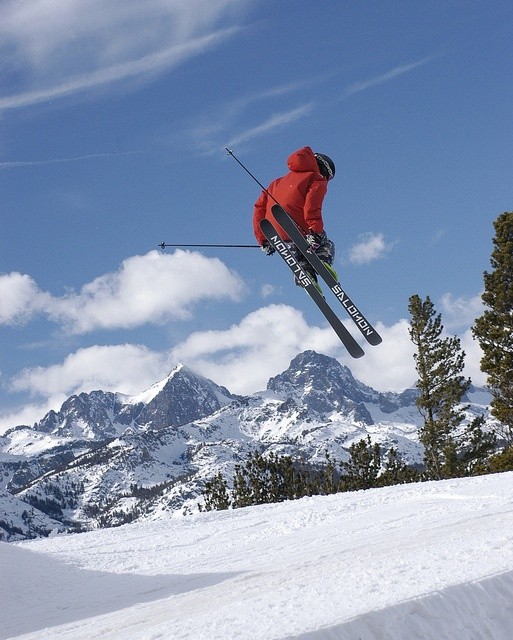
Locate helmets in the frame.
[313,153,335,180]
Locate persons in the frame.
[252,146,337,298]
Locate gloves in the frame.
[260,243,275,256]
[305,232,324,255]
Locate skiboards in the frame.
[260,205,382,359]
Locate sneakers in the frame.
[320,259,338,283]
[304,271,326,300]
